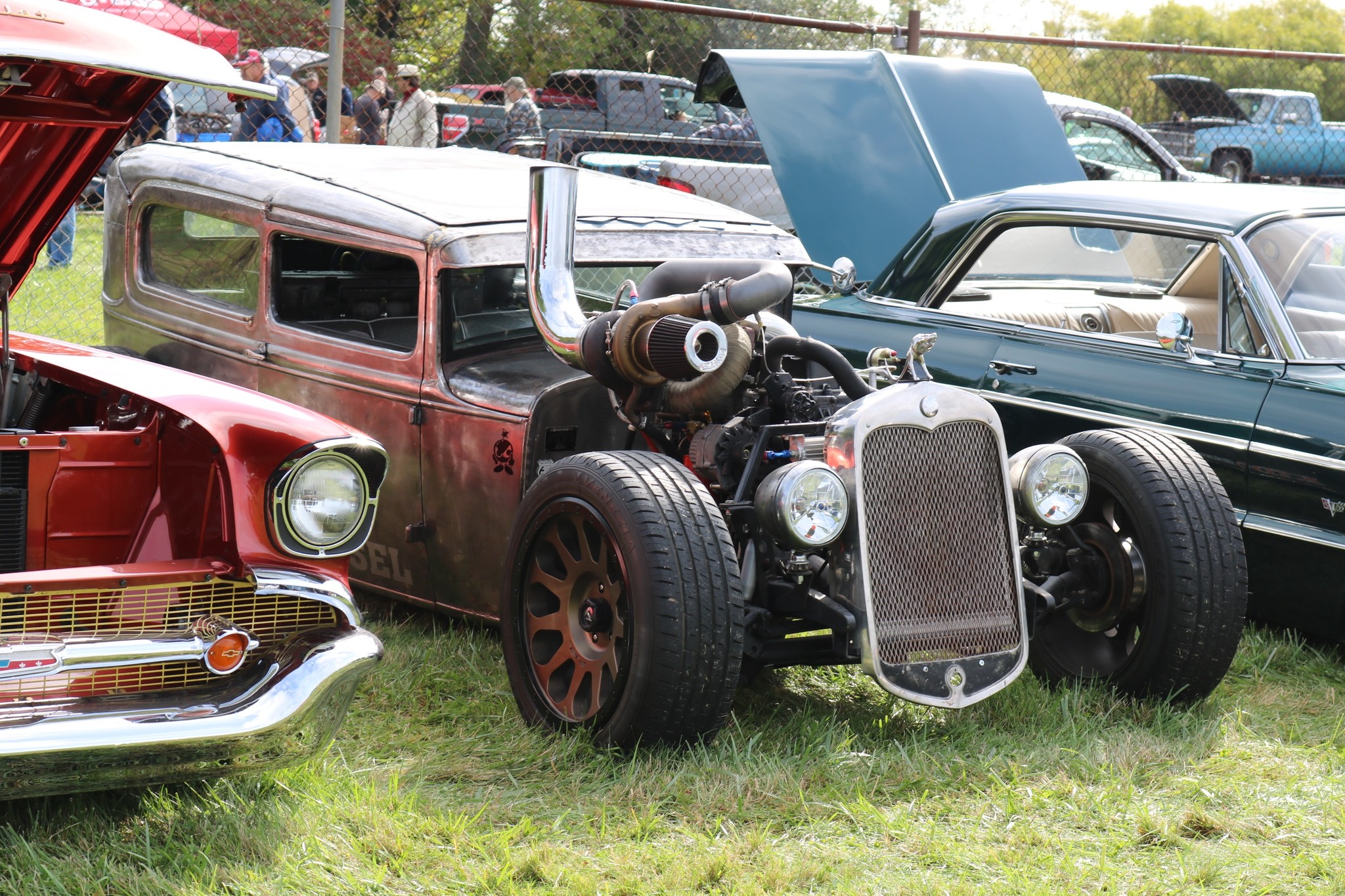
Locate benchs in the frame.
[964,296,1221,341]
[276,262,536,350]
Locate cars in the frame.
[169,45,330,116]
[566,49,1345,556]
[440,83,538,105]
[102,139,1246,779]
[1,1,390,804]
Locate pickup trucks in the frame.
[1138,73,1345,181]
[385,68,768,166]
[539,92,1234,296]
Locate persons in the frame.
[1120,106,1133,117]
[503,76,543,158]
[304,71,327,128]
[123,84,178,150]
[354,80,386,145]
[386,64,439,148]
[227,48,316,142]
[341,80,353,116]
[1172,111,1184,121]
[40,201,76,270]
[372,67,397,124]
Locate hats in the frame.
[306,71,318,81]
[232,48,264,69]
[502,76,526,89]
[365,79,386,94]
[394,64,420,77]
